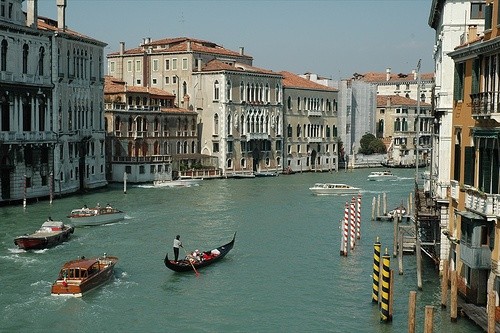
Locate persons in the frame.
[47,217,53,222]
[82,204,89,211]
[106,203,112,212]
[193,249,212,262]
[173,235,183,263]
[97,203,101,208]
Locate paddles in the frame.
[181,245,200,277]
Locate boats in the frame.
[308,183,360,197]
[232,172,256,178]
[13,220,75,252]
[163,231,237,272]
[254,170,280,176]
[64,206,126,228]
[385,200,406,216]
[153,178,204,187]
[50,253,120,297]
[368,172,398,182]
